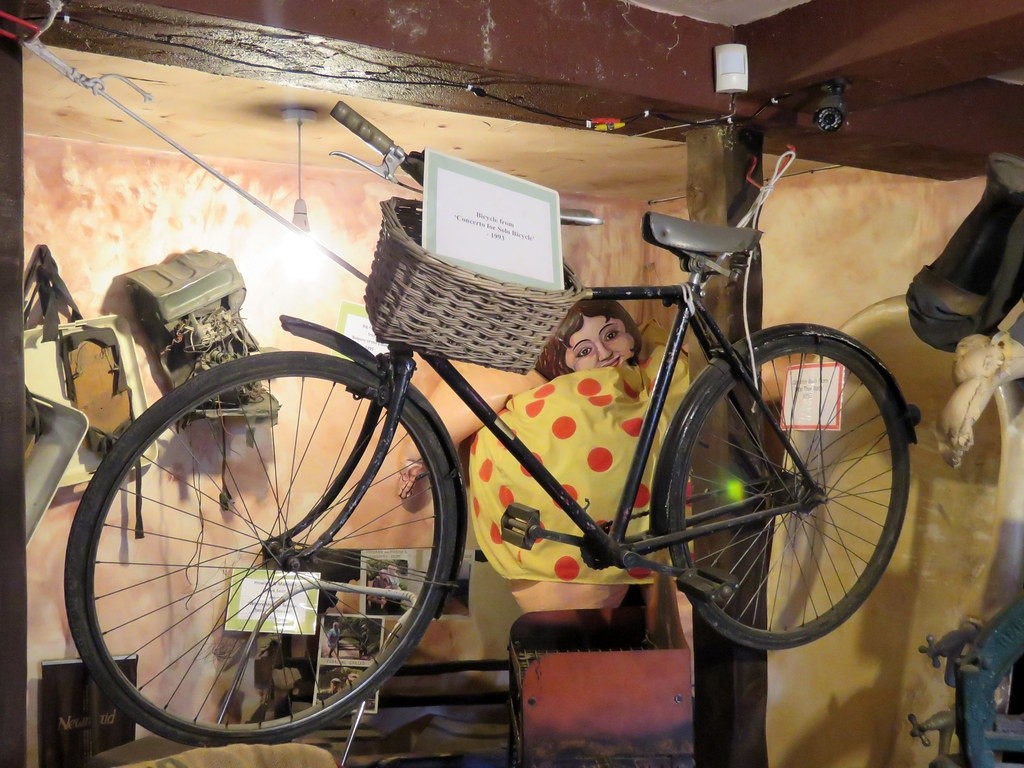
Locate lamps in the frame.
[281,109,317,231]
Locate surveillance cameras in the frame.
[813,84,852,133]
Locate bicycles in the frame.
[64,101,921,749]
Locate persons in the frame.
[367,564,402,608]
[327,621,340,658]
[330,672,359,693]
[360,624,370,659]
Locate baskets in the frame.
[364,196,587,376]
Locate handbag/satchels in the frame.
[24,244,159,490]
